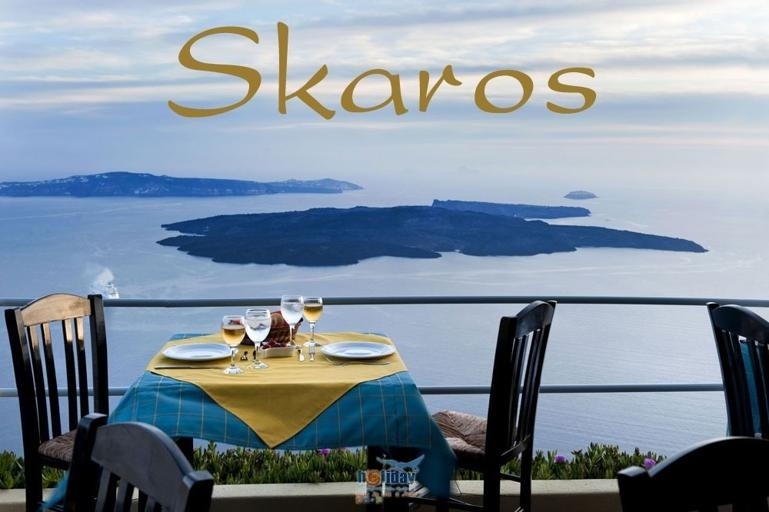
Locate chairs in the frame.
[617,301,769,512]
[4,293,214,512]
[386,300,557,512]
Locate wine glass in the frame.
[221,296,323,375]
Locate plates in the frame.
[163,342,233,361]
[320,342,395,358]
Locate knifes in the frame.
[154,365,223,369]
